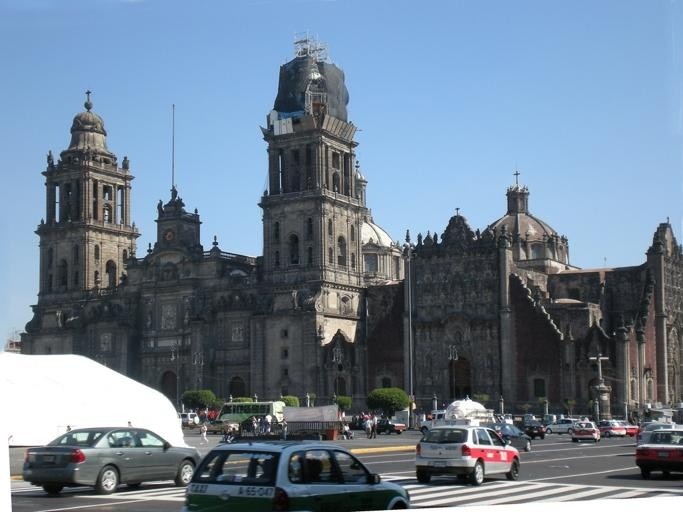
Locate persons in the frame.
[200,422,208,445]
[66,426,71,433]
[219,427,238,443]
[260,417,271,437]
[128,421,132,427]
[341,410,378,439]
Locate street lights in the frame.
[402,241,417,432]
[171,344,179,406]
[193,352,206,389]
[332,345,344,395]
[448,345,459,403]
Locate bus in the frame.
[216,401,286,424]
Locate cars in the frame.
[416,410,683,487]
[200,419,239,435]
[182,440,409,512]
[23,427,201,494]
[377,419,406,435]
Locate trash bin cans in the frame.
[326,428,336,440]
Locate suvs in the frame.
[179,413,200,430]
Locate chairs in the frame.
[242,458,325,481]
